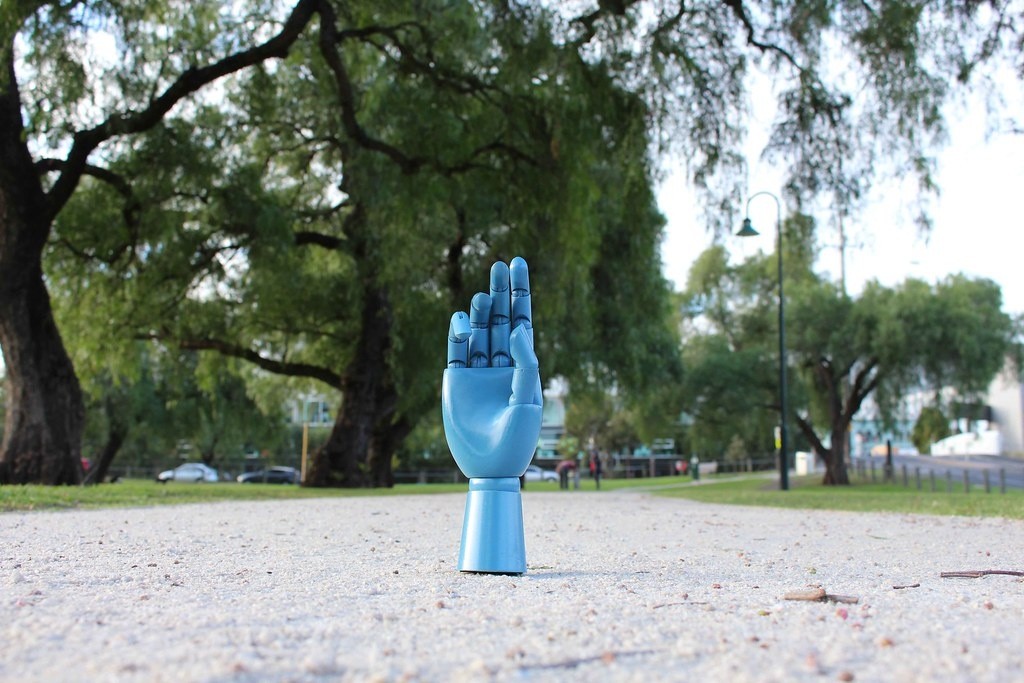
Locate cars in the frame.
[158,462,219,483]
[236,465,296,484]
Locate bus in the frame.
[524,464,560,484]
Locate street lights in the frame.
[734,190,791,490]
[296,388,319,481]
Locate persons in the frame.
[592,450,603,490]
[440,256,543,575]
